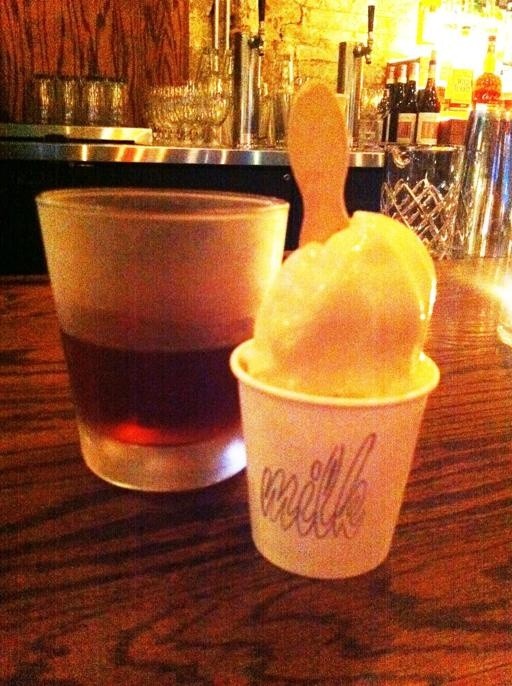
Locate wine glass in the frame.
[137,78,235,149]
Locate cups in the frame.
[37,187,291,490]
[32,78,135,131]
[230,333,440,584]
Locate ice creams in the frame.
[230,212,439,581]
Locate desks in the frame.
[2,255,512,686]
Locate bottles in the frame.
[377,38,511,258]
